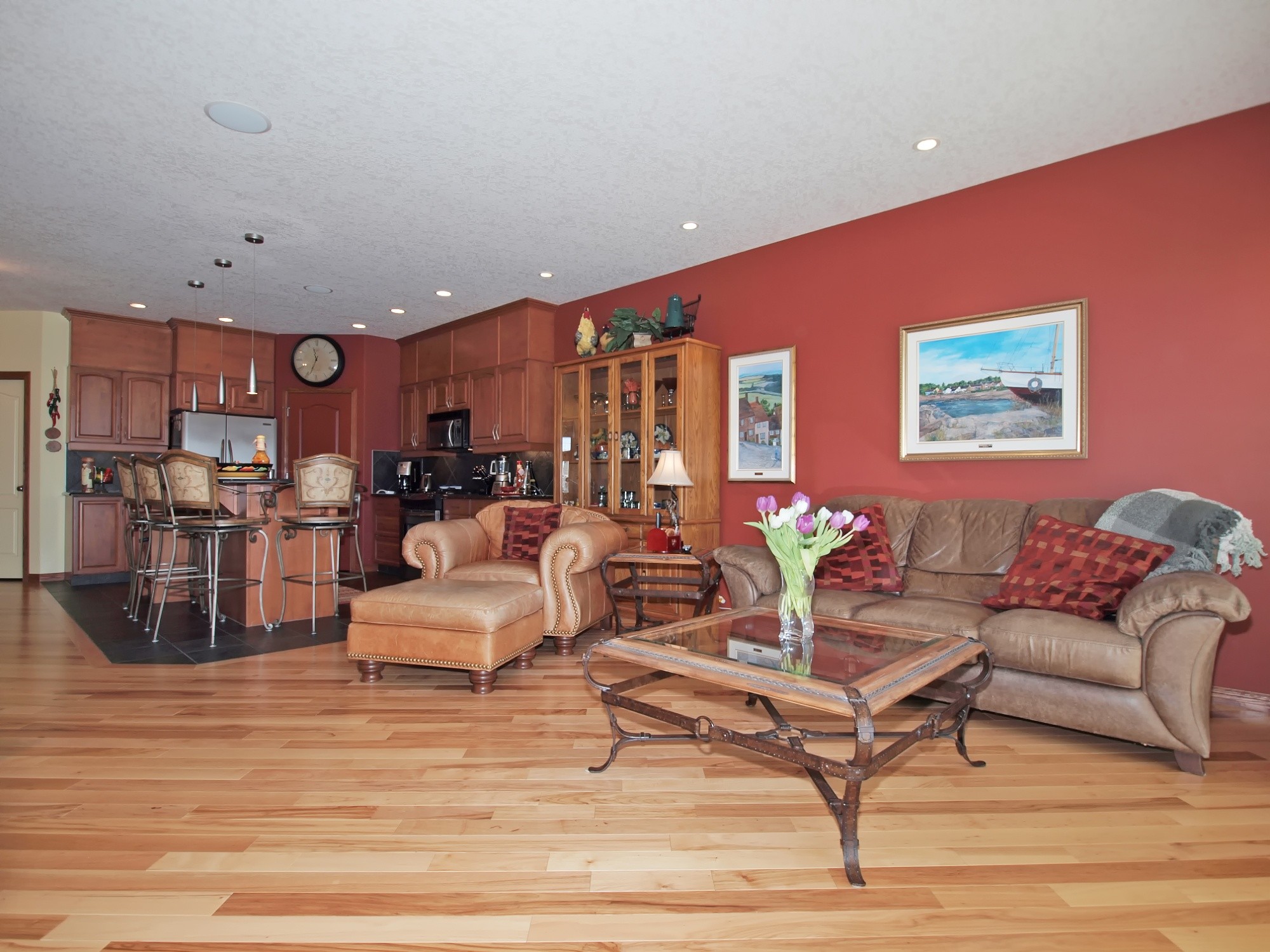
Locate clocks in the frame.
[290,335,345,388]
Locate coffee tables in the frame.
[578,604,993,889]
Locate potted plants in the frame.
[604,308,665,355]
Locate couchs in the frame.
[402,499,632,656]
[714,496,1249,780]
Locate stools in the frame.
[151,449,274,649]
[343,577,546,698]
[113,456,206,616]
[272,453,368,635]
[126,454,226,631]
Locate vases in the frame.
[771,559,817,644]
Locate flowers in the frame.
[743,489,870,624]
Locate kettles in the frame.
[421,473,433,493]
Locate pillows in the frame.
[495,504,564,564]
[796,502,900,592]
[983,511,1172,620]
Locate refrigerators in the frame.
[169,408,279,478]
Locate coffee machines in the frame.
[396,460,420,493]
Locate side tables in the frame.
[600,543,724,637]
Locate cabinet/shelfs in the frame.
[392,298,553,456]
[552,337,725,626]
[69,493,141,582]
[64,363,170,456]
[373,496,403,568]
[170,370,276,416]
[441,499,504,521]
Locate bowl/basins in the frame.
[501,486,515,494]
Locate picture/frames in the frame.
[725,344,796,485]
[896,297,1089,461]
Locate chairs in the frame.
[656,295,702,342]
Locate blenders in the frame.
[489,454,511,496]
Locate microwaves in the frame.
[425,408,471,449]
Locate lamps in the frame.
[646,448,696,551]
[185,280,204,410]
[242,232,264,395]
[213,256,231,406]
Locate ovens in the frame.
[399,506,444,553]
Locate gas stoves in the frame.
[400,488,463,510]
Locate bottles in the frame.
[80,456,107,494]
[515,460,531,496]
